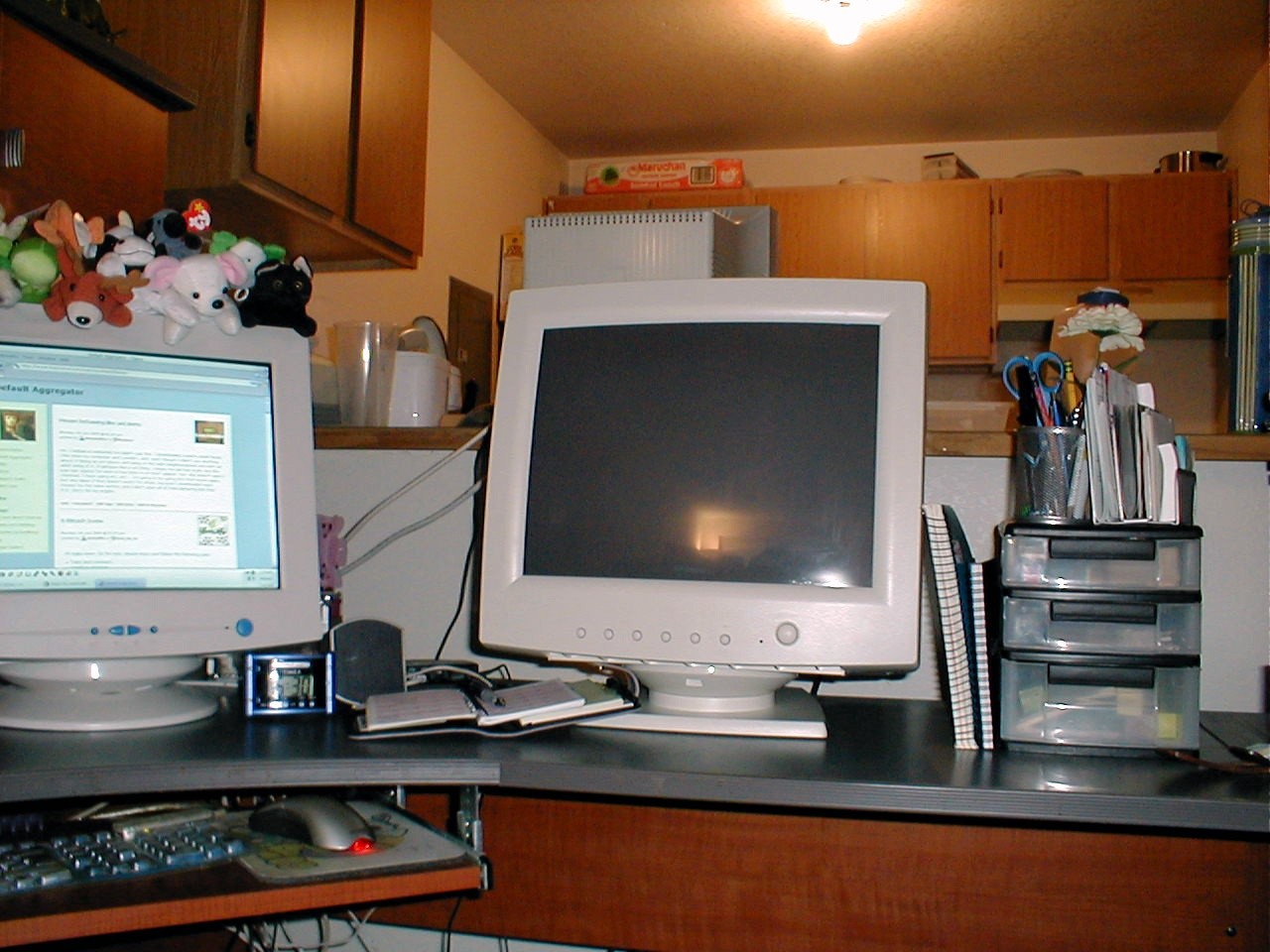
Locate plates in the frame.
[839,176,892,183]
[1014,168,1082,176]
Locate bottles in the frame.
[1225,198,1270,437]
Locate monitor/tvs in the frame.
[478,281,929,740]
[1,301,324,729]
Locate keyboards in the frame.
[1,822,247,899]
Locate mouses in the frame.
[250,795,376,853]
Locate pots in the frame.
[1153,150,1230,173]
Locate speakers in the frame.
[332,619,407,709]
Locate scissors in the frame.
[1002,351,1070,491]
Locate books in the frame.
[966,557,1000,751]
[919,503,982,751]
[348,678,643,737]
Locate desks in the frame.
[0,670,1270,950]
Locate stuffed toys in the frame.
[1,199,318,345]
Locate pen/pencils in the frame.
[469,679,505,707]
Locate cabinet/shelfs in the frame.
[547,186,755,211]
[998,527,1204,758]
[92,0,431,269]
[756,180,999,367]
[994,172,1239,321]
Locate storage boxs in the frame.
[584,157,744,192]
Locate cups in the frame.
[390,350,451,427]
[330,320,399,426]
